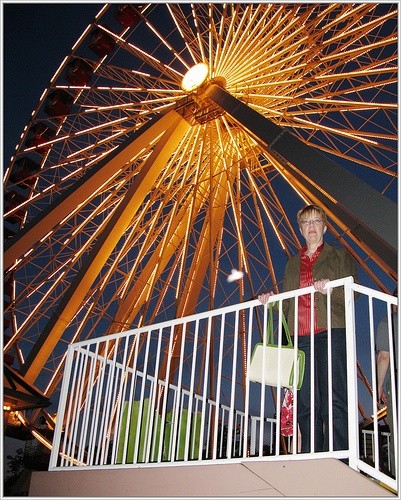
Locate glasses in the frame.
[301,218,323,225]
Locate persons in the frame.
[375,285,398,480]
[255,203,360,466]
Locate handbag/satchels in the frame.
[249,296,305,390]
